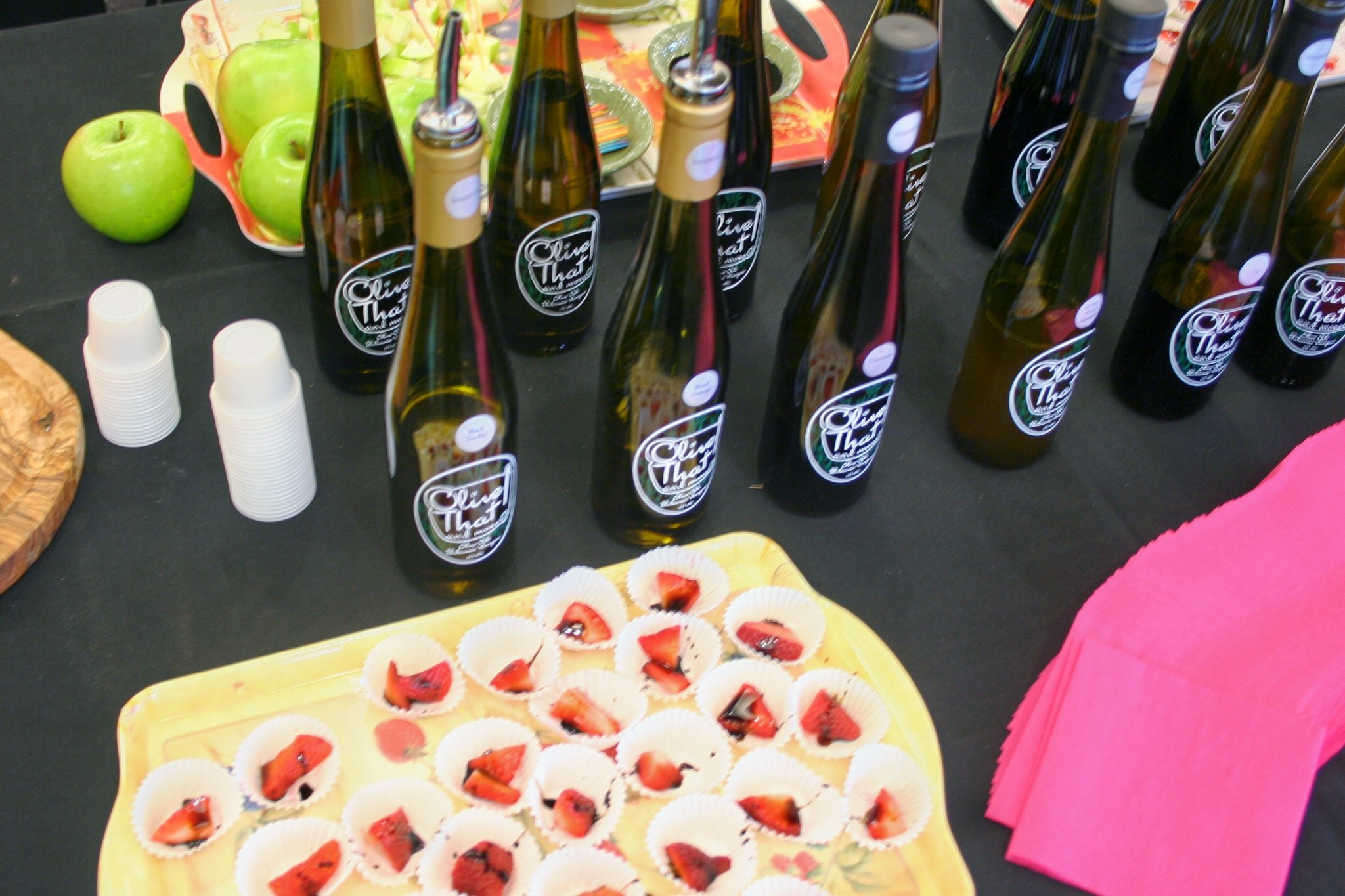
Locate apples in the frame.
[62,110,195,240]
[217,0,510,243]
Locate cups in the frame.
[208,317,316,521]
[486,71,653,176]
[645,18,803,103]
[83,279,183,449]
[577,0,661,22]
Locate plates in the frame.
[159,0,852,257]
[97,532,978,896]
[987,0,1345,123]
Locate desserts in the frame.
[132,545,929,896]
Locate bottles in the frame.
[714,1,773,317]
[946,0,1169,471]
[489,0,604,356]
[591,3,736,541]
[963,1,1101,249]
[812,0,942,256]
[383,10,519,591]
[302,1,416,393]
[757,15,940,517]
[1110,1,1345,421]
